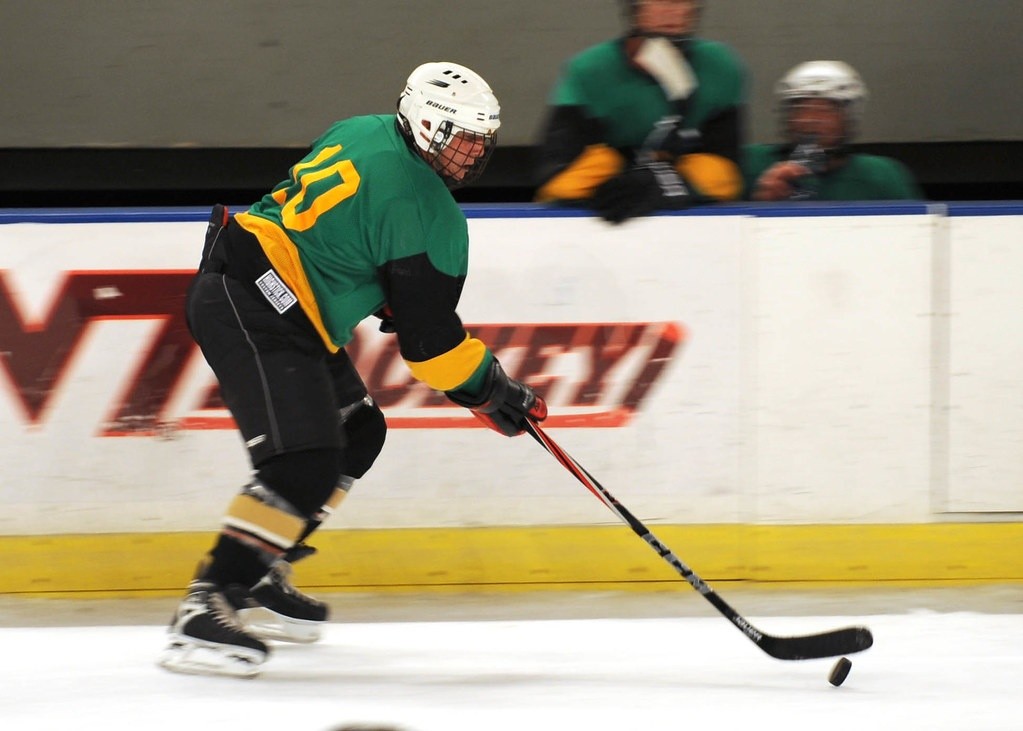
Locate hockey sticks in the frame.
[615,28,701,162]
[494,403,873,661]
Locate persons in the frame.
[726,59,918,201]
[526,2,749,222]
[151,62,549,678]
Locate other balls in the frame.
[829,657,852,687]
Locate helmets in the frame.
[395,61,502,189]
[777,59,867,101]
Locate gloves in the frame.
[443,357,548,437]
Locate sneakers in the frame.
[157,576,270,678]
[234,561,330,645]
